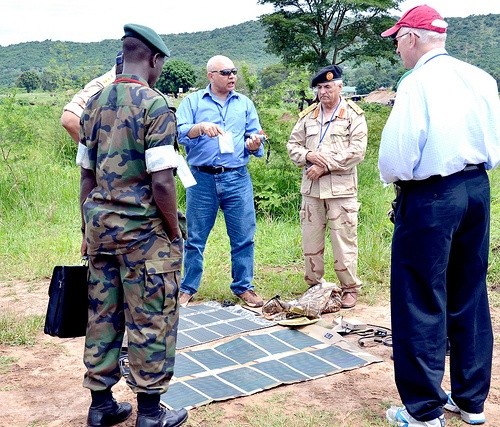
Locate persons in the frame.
[286,65,364,308]
[174,55,265,308]
[60,51,130,379]
[77,22,188,427]
[378,4,500,427]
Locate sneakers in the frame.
[442,391,486,424]
[237,290,264,307]
[387,406,446,427]
[177,291,194,306]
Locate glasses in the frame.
[391,32,420,46]
[212,68,236,76]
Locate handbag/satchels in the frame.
[43,259,89,338]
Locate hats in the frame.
[121,23,171,57]
[311,66,344,88]
[381,5,448,37]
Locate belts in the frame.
[193,165,240,174]
[454,165,478,173]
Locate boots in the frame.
[87,387,133,427]
[136,393,188,427]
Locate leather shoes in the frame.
[339,292,357,308]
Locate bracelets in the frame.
[199,123,204,136]
